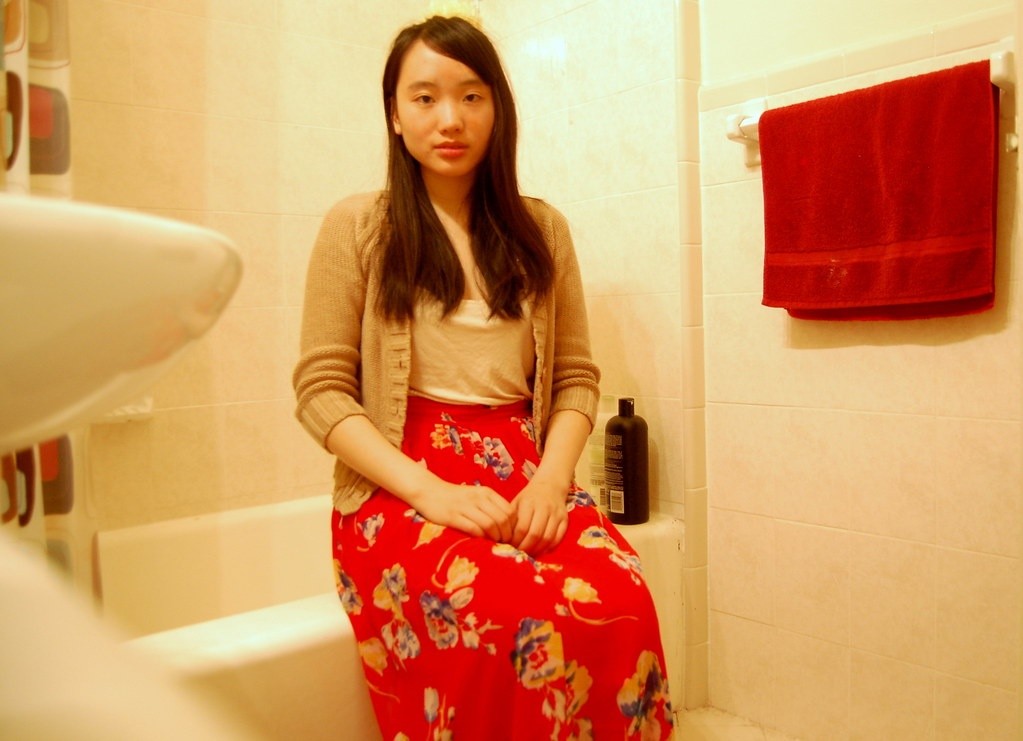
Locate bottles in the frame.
[605,399,649,525]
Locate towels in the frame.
[756,56,1000,319]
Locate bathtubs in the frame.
[87,494,690,741]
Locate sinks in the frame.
[0,188,243,459]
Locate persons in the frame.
[291,17,676,741]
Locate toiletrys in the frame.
[587,392,652,526]
